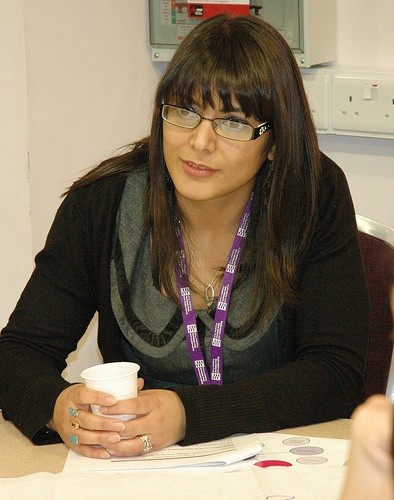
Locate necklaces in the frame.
[172,190,255,386]
[191,248,231,309]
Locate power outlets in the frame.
[299,73,329,130]
[332,75,393,135]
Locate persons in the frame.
[0,13,369,460]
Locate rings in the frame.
[69,429,81,445]
[68,406,82,429]
[138,434,152,453]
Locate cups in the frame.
[80,362,140,439]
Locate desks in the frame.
[1,409,357,500]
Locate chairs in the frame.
[354,213,394,404]
